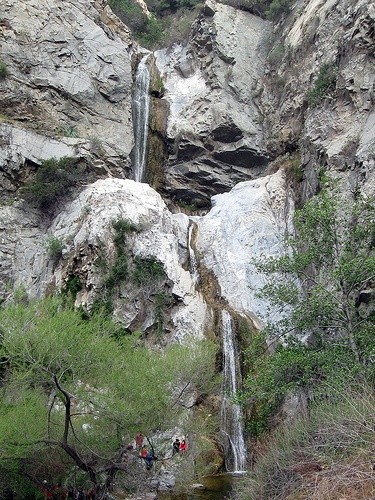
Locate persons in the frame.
[41,479,67,500]
[179,440,186,456]
[173,439,179,456]
[136,430,156,470]
